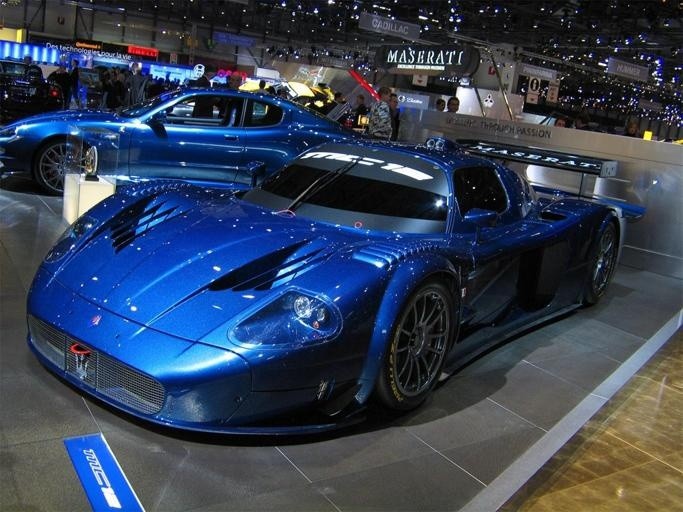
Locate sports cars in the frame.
[0,87,368,197]
[237,79,335,109]
[0,60,63,123]
[291,96,359,128]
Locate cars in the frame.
[25,137,647,437]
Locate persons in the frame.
[620,118,640,138]
[553,115,571,128]
[573,111,592,131]
[25,56,461,146]
